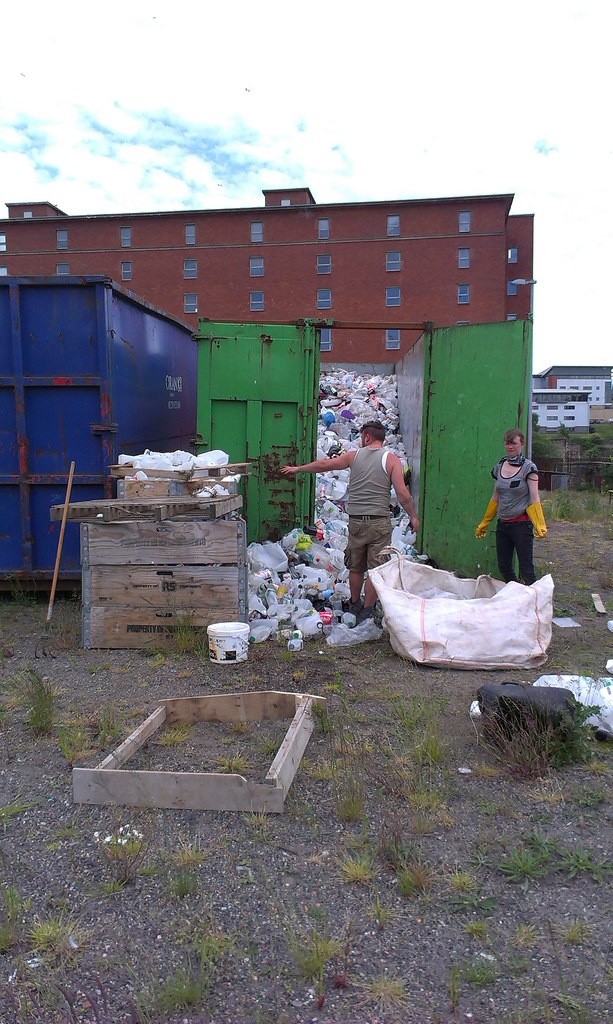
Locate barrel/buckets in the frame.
[207,622,251,664]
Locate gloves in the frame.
[526,502,547,539]
[475,498,499,539]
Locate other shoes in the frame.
[357,602,384,623]
[347,597,364,615]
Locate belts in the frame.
[349,515,386,520]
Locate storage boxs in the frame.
[78,523,249,650]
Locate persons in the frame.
[473,429,549,585]
[279,420,419,613]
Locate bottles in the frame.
[114,367,421,651]
